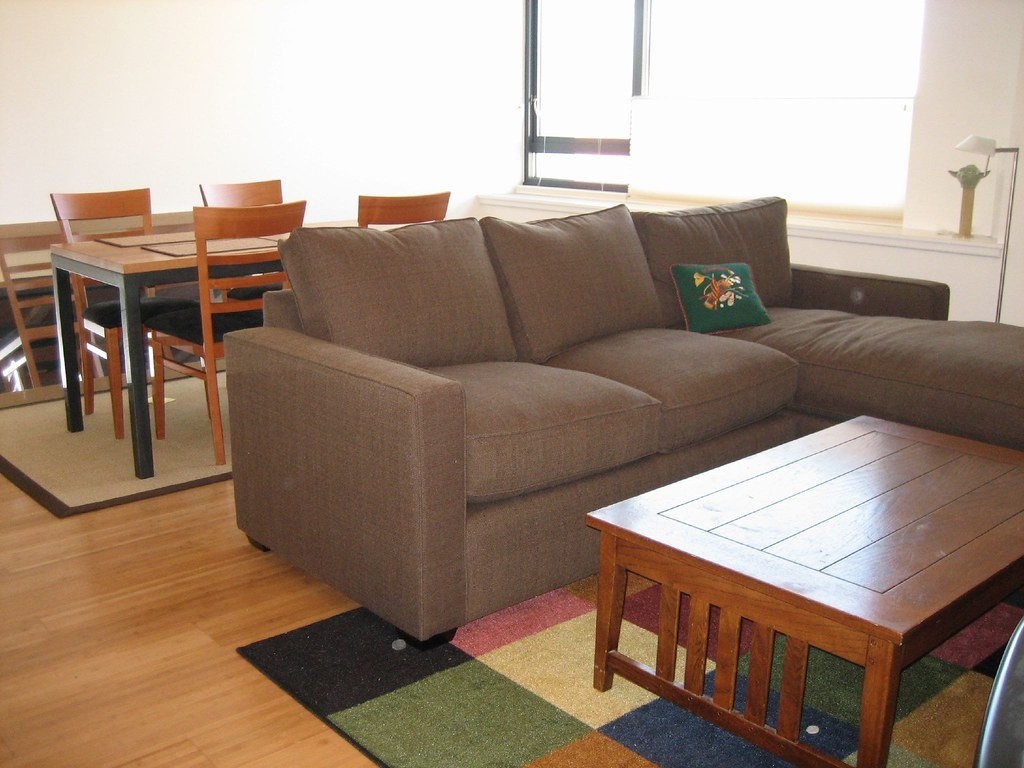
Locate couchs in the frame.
[222,194,1024,648]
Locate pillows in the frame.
[671,260,772,335]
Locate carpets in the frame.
[234,566,1024,766]
[0,371,232,520]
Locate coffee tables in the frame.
[584,410,1024,768]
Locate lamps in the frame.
[954,134,1019,323]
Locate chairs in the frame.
[50,188,202,441]
[356,192,450,231]
[197,180,287,303]
[0,232,105,390]
[141,201,306,465]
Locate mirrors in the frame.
[0,211,227,410]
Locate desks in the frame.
[50,220,359,482]
